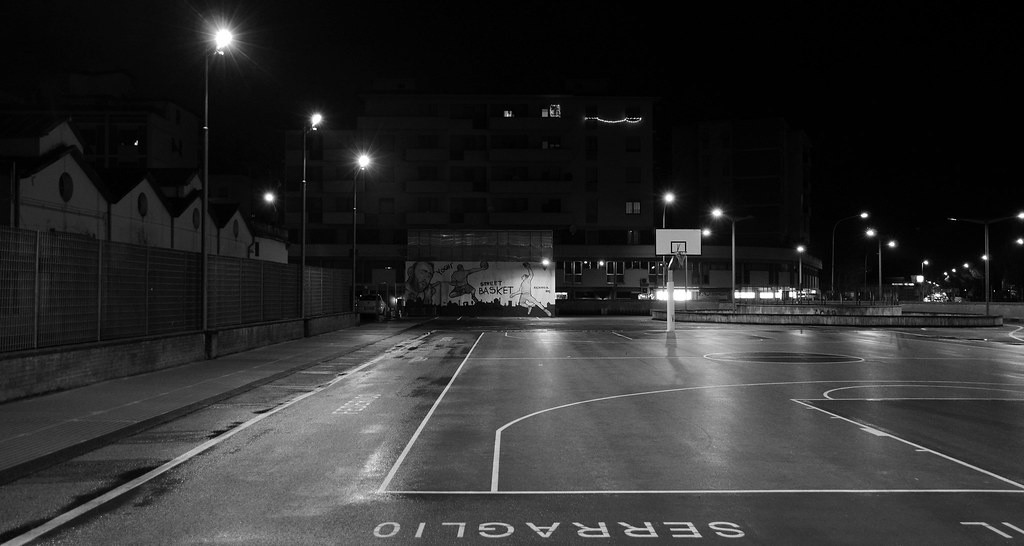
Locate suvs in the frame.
[357,293,387,317]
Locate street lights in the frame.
[867,229,896,302]
[684,226,711,298]
[831,212,868,297]
[797,245,804,303]
[662,193,674,290]
[922,260,929,301]
[712,209,736,310]
[353,155,373,309]
[301,112,326,318]
[947,211,1024,316]
[200,27,233,330]
